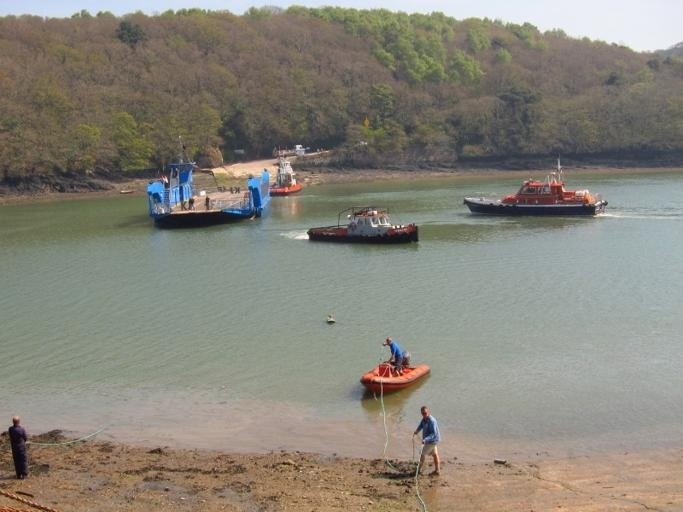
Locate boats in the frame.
[463,153,608,216]
[269,156,302,195]
[359,361,431,393]
[307,206,419,244]
[146,158,270,224]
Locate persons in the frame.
[409,406,441,476]
[382,336,404,374]
[7,415,29,479]
